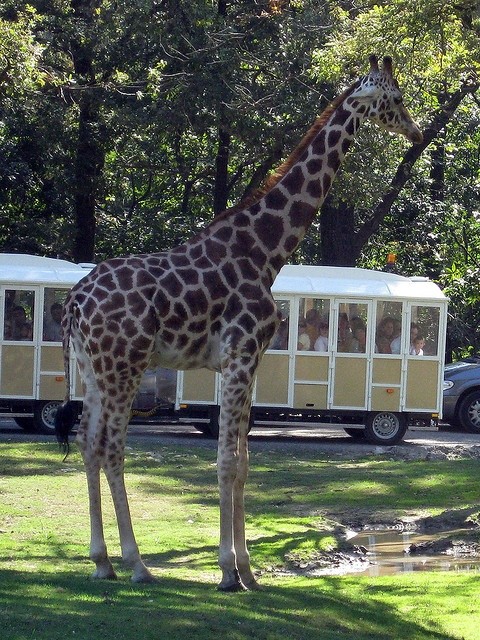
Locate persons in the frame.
[13,307,33,339]
[4,290,17,338]
[46,304,65,341]
[20,323,31,339]
[267,308,425,356]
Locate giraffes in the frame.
[55,52,423,592]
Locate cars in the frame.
[443,353,480,433]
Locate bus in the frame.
[0,252,450,444]
